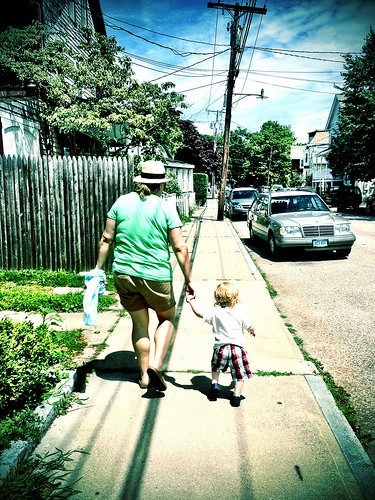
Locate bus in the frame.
[219,179,232,191]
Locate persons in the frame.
[186,282,255,405]
[95,160,196,391]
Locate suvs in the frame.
[321,185,362,216]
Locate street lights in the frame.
[217,89,269,222]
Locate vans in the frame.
[225,187,259,219]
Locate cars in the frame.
[259,183,313,193]
[365,197,375,216]
[246,189,357,260]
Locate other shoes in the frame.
[209,383,221,396]
[229,394,242,407]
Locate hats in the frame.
[134,160,172,183]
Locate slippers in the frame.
[139,376,148,388]
[146,365,167,391]
